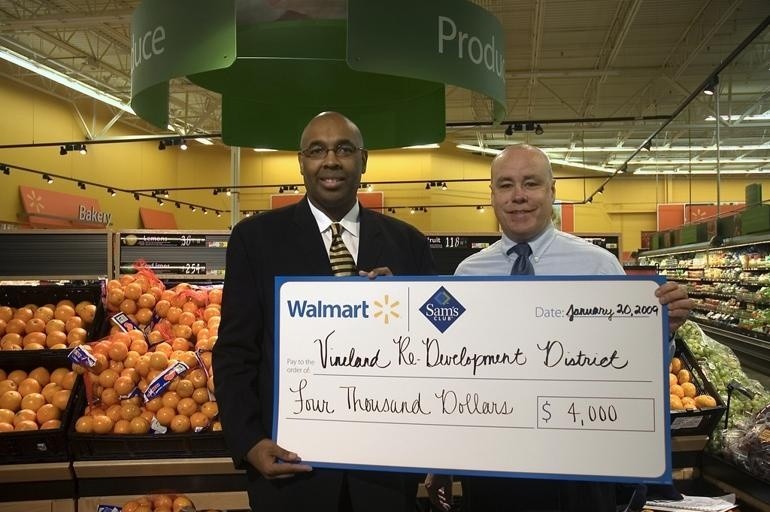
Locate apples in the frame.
[122,494,195,512]
[1,267,223,435]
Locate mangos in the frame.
[668,358,718,412]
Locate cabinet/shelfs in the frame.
[637,233,770,376]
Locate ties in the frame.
[330,223,357,277]
[508,245,535,275]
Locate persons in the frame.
[212,110,437,512]
[360,144,693,511]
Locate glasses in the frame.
[300,144,364,160]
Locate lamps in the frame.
[355,121,653,215]
[708,77,726,248]
[703,85,715,95]
